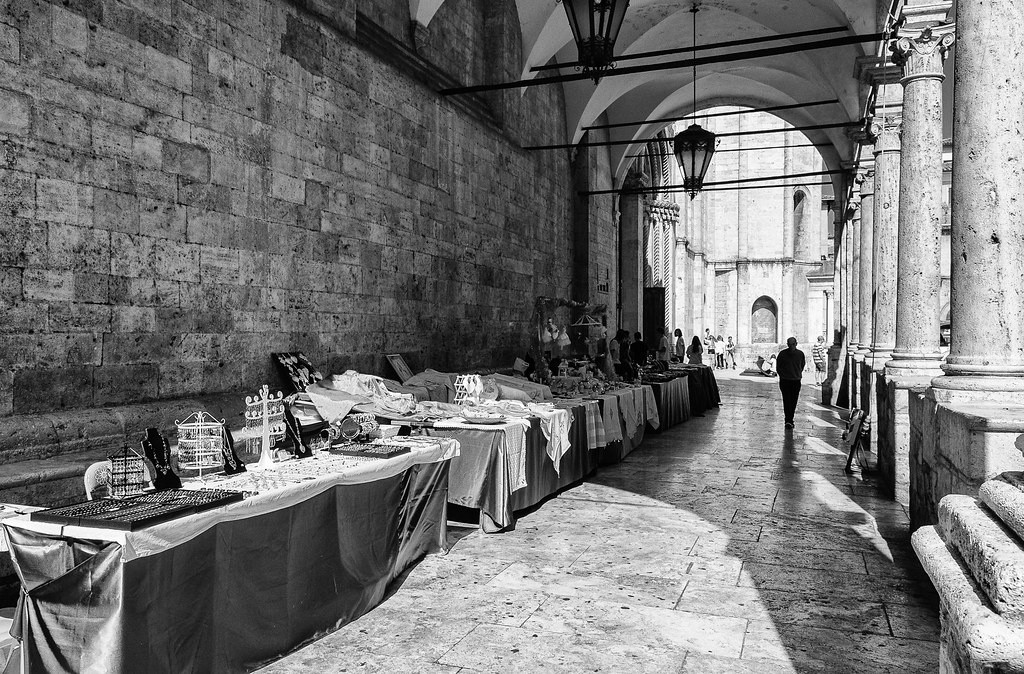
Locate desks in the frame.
[0,355,722,674]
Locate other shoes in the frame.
[786,422,794,427]
[816,382,822,386]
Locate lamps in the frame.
[558,1,630,85]
[664,5,721,202]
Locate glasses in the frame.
[789,343,796,345]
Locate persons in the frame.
[609,328,737,376]
[776,337,806,429]
[764,354,777,376]
[812,336,826,386]
